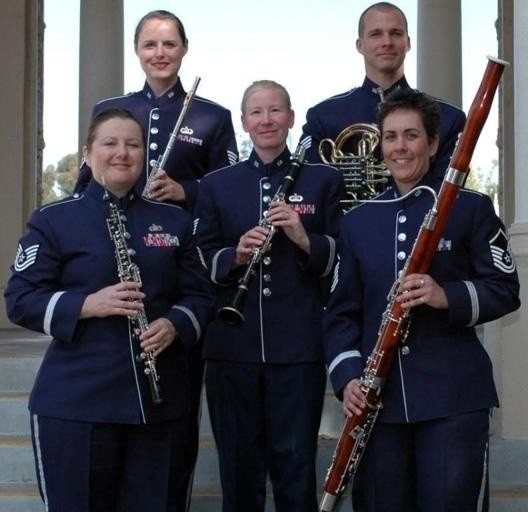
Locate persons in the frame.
[71,10,239,216]
[295,2,469,212]
[4,105,211,511]
[319,89,520,511]
[188,80,345,511]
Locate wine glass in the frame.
[420,279,424,288]
[420,296,425,304]
